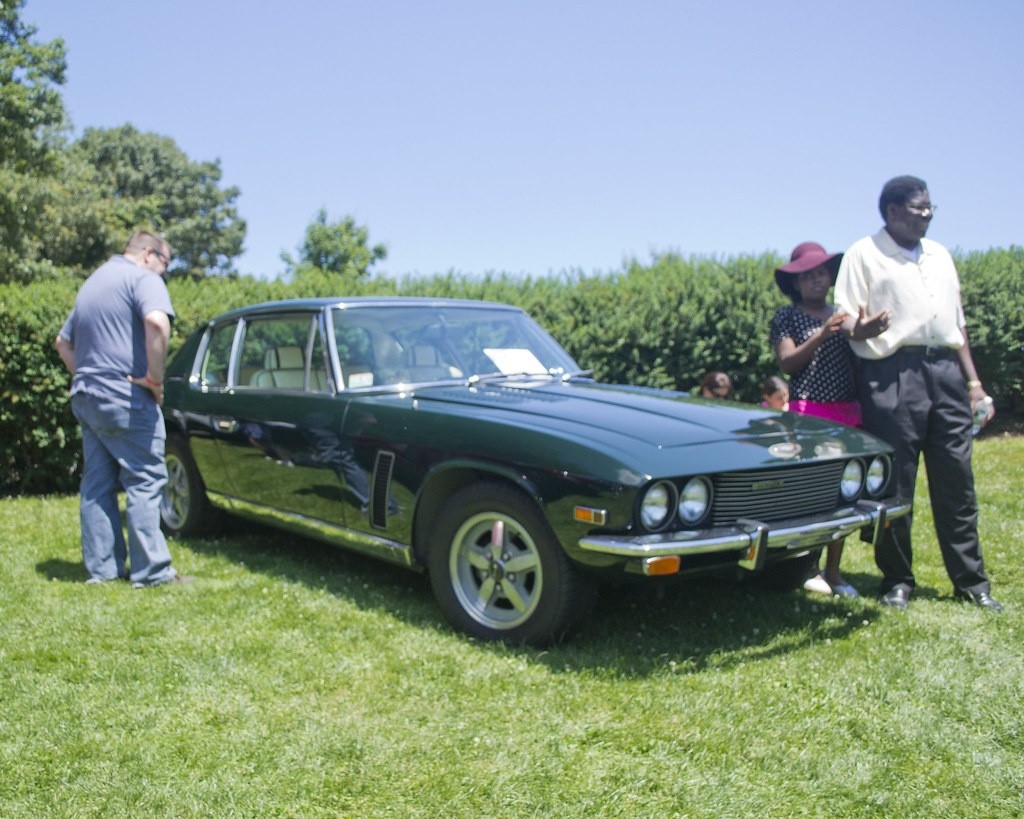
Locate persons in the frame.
[771,242,863,598]
[757,376,790,412]
[56,229,196,588]
[701,371,731,399]
[835,175,1005,615]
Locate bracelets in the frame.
[966,380,981,390]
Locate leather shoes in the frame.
[968,591,1003,610]
[876,589,912,610]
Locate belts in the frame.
[898,346,959,359]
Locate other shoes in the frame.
[831,584,860,598]
[801,573,832,595]
[166,574,195,584]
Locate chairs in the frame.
[248,346,329,392]
[394,344,451,383]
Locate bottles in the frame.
[972,396,992,434]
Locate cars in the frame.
[155,294,916,653]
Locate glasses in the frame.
[142,247,172,269]
[899,202,936,215]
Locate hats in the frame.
[774,242,845,300]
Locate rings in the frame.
[879,326,885,332]
